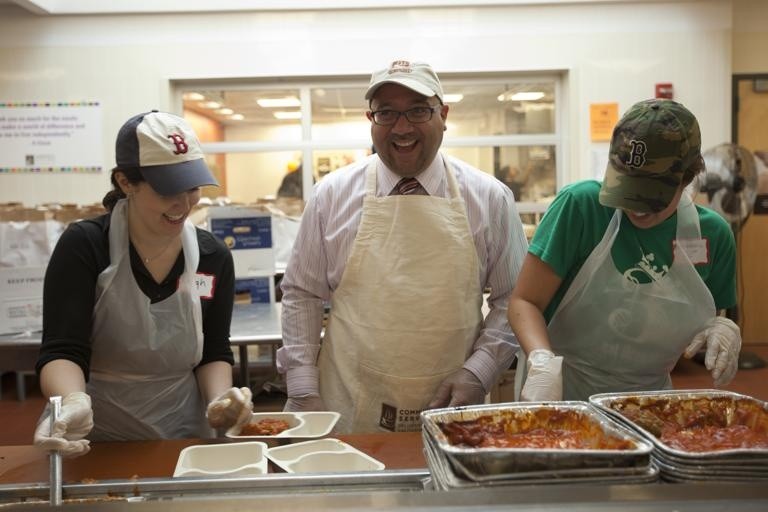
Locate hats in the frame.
[363,60,444,104]
[597,97,702,213]
[115,110,220,197]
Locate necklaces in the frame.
[127,232,174,263]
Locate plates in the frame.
[419,400,660,491]
[589,388,768,484]
[0,468,430,512]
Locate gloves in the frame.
[204,387,254,427]
[33,393,94,460]
[428,369,485,408]
[520,350,564,402]
[684,317,742,387]
[283,395,324,412]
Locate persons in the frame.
[506,98,742,400]
[276,57,527,432]
[33,109,255,457]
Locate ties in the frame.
[397,178,417,195]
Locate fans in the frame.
[691,143,766,374]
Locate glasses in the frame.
[369,107,437,125]
